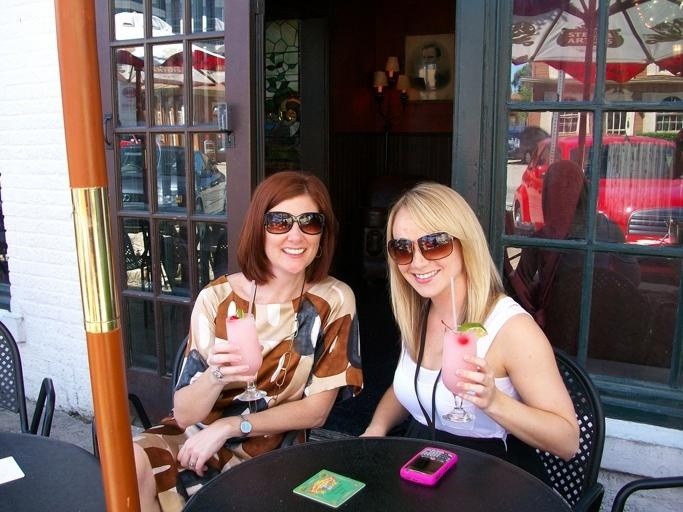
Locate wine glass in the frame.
[438,329,477,425]
[225,312,269,402]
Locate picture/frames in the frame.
[400,32,454,104]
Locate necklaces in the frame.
[245,267,308,438]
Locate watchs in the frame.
[238,414,253,439]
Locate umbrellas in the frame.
[510,0,683,171]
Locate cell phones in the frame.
[644,241,661,246]
[400,446,459,487]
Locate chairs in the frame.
[0,322,55,436]
[122,210,227,333]
[545,267,649,363]
[405,346,605,512]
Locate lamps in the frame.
[365,53,410,185]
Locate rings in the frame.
[190,464,196,467]
[213,366,224,379]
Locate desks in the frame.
[0,435,108,510]
[182,436,572,511]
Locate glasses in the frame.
[387,231,458,265]
[263,211,326,235]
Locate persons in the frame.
[132,170,365,512]
[358,180,580,489]
[504,160,642,359]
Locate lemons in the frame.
[457,322,487,342]
[236,308,244,319]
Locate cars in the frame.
[506,123,550,163]
[512,132,682,284]
[118,144,226,254]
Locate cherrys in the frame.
[441,320,469,345]
[230,316,238,320]
